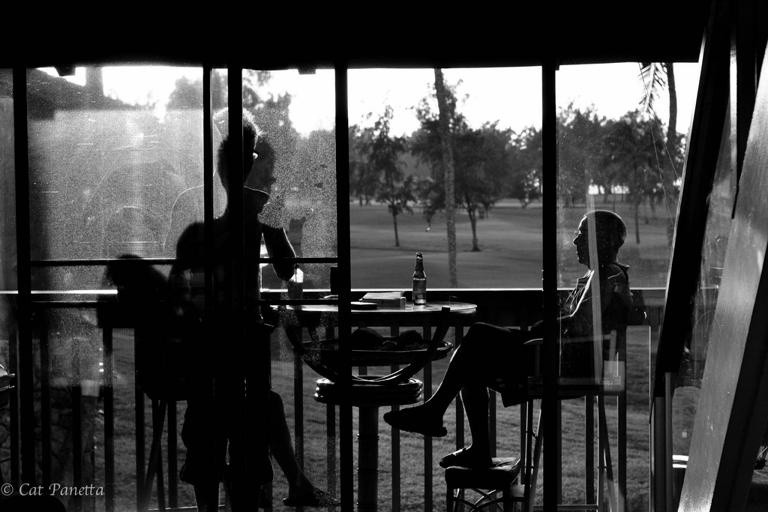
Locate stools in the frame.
[445,455,520,511]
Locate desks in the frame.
[271,302,477,512]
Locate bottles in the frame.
[410,252,427,306]
[287,265,304,309]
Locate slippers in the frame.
[439,448,496,468]
[283,487,341,506]
[384,406,447,437]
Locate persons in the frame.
[383,209,629,470]
[180,390,341,512]
[164,108,298,283]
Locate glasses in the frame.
[574,227,597,237]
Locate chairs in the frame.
[520,263,644,509]
